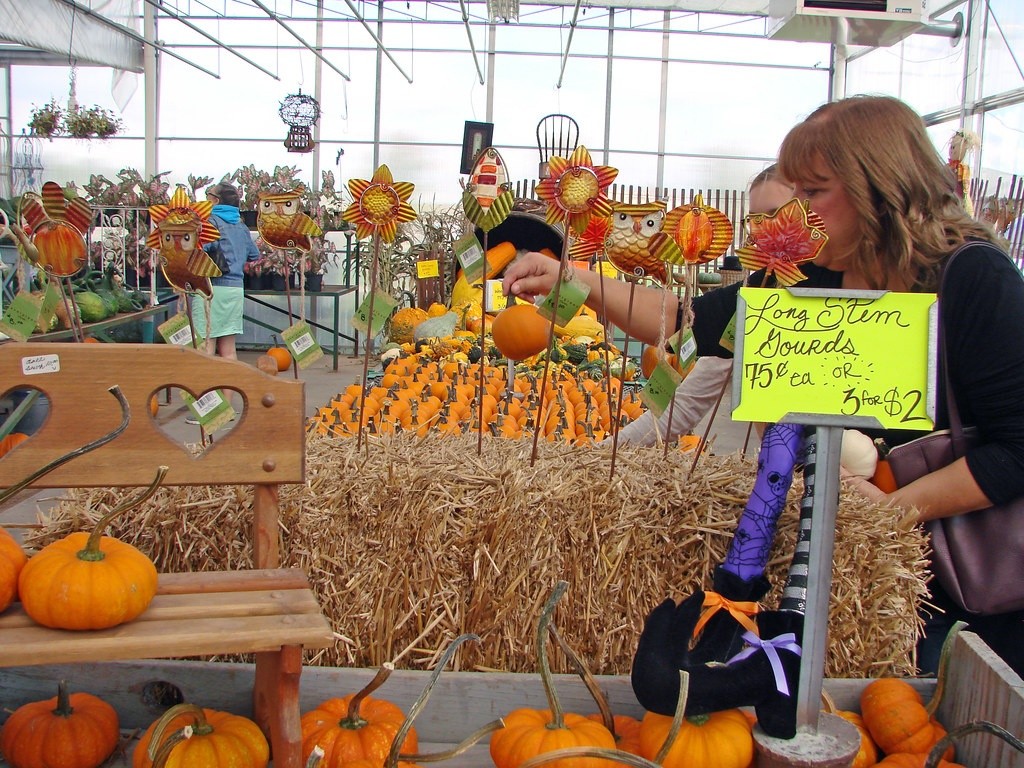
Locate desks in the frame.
[0,304,170,342]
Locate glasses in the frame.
[207,189,220,199]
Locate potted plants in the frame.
[79,166,344,292]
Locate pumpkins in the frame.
[0,195,149,344]
[132,579,1024,768]
[0,523,28,614]
[266,334,291,370]
[19,466,170,630]
[0,680,119,768]
[151,395,159,417]
[304,240,710,454]
[0,431,32,459]
[838,427,897,492]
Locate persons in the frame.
[501,93,1024,679]
[596,161,797,449]
[184,182,262,425]
[942,128,982,219]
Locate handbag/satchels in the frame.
[203,217,231,274]
[884,240,1024,616]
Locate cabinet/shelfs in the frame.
[132,227,360,372]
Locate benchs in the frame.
[0,341,334,768]
